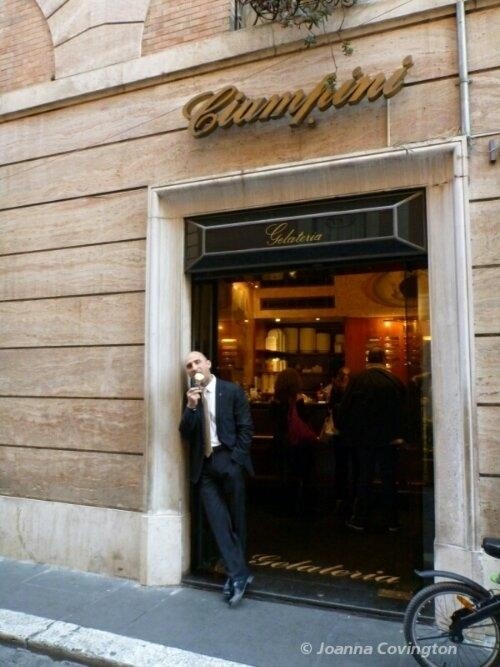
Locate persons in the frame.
[177,349,258,609]
[266,340,429,538]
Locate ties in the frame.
[202,389,212,458]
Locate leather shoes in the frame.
[223,574,254,606]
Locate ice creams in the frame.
[194,373,206,388]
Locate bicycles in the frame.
[400,535,500,667]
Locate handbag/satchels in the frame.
[285,399,318,448]
